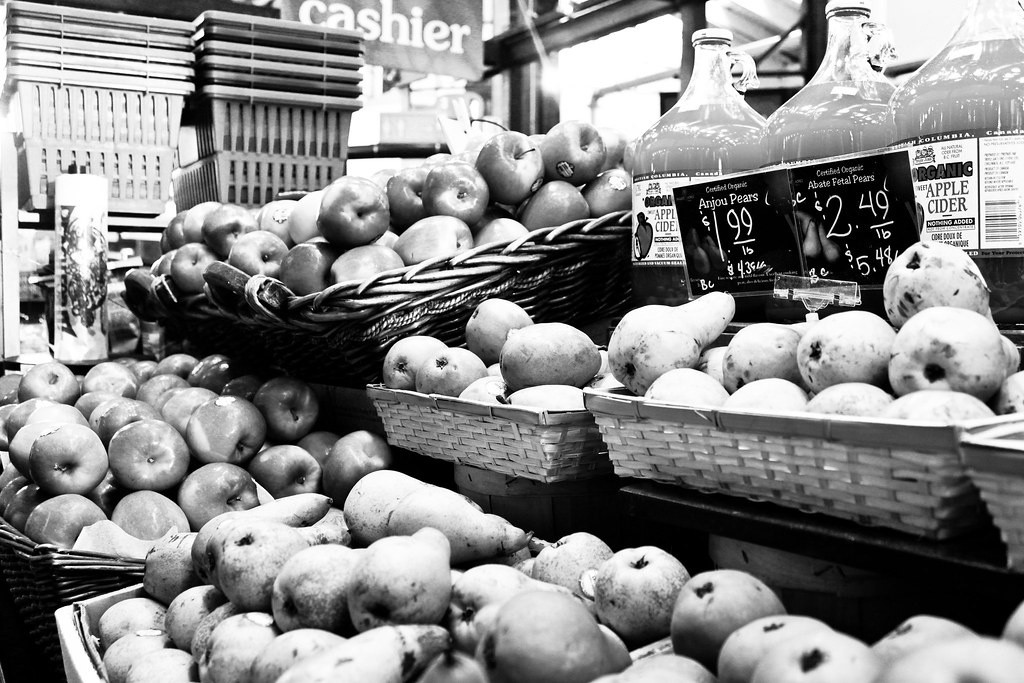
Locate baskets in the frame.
[956,416,1024,573]
[0,506,148,655]
[366,380,612,484]
[142,208,671,389]
[581,350,1024,542]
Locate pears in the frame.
[380,237,1024,428]
[91,470,1024,683]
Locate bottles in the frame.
[766,0,899,322]
[884,0,1024,324]
[630,27,767,305]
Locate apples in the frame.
[0,121,637,552]
